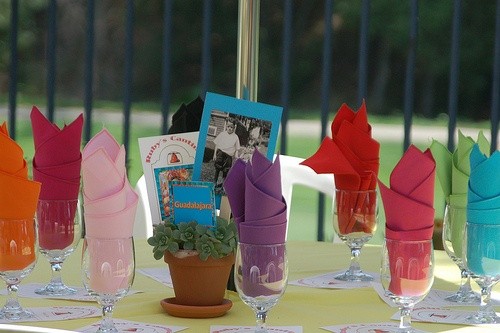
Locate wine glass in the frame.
[332,189,379,282]
[380,238,435,333]
[0,218,37,320]
[34,199,83,296]
[442,205,491,304]
[234,242,289,333]
[80,235,136,333]
[462,221,500,324]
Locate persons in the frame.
[212,117,258,193]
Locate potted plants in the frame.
[147,216,239,318]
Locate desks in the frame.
[0,238,500,333]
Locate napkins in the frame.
[298,97,380,234]
[81,129,139,293]
[222,148,288,298]
[429,129,500,275]
[30,105,84,249]
[0,121,42,272]
[166,96,204,135]
[365,144,436,296]
